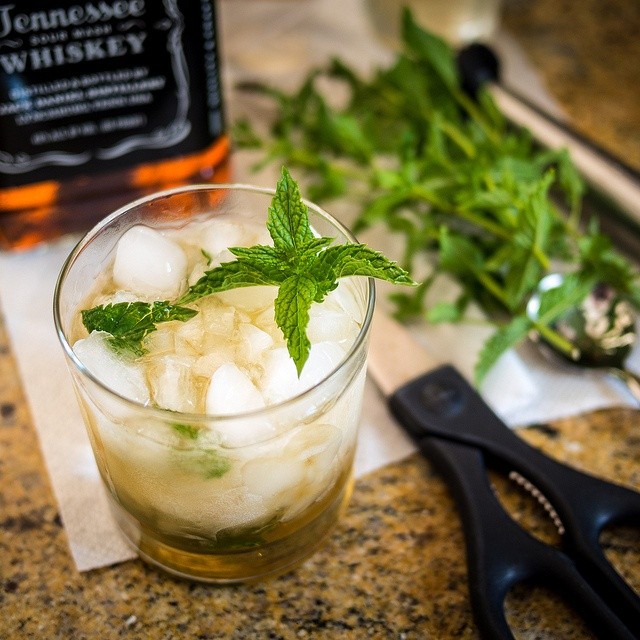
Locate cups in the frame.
[49,182,377,587]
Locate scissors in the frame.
[362,309,640,640]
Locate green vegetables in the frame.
[232,4,636,388]
